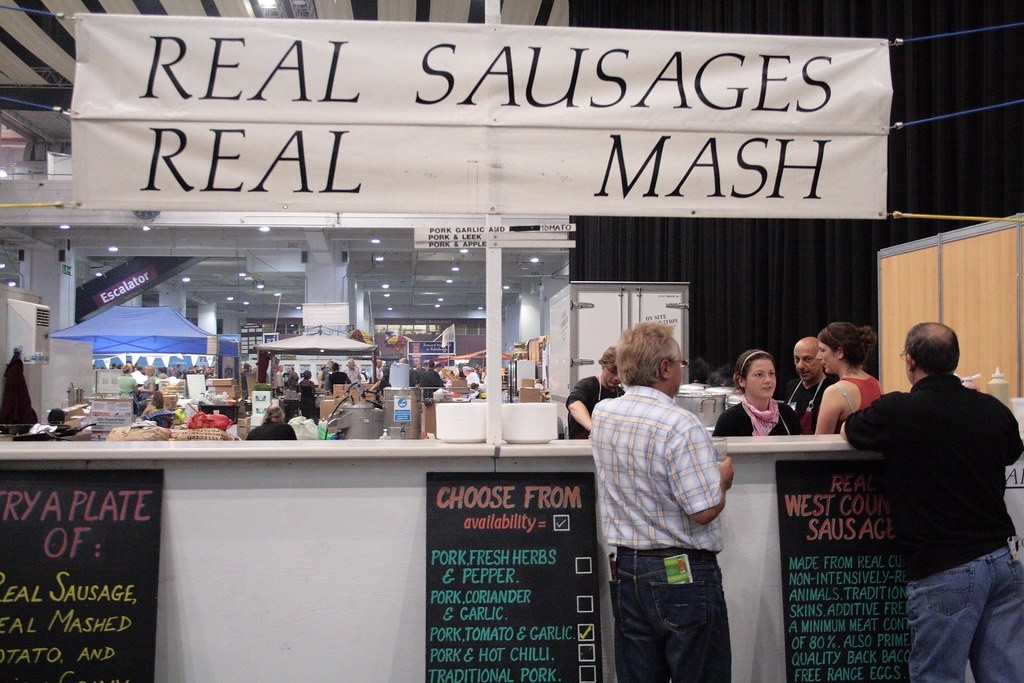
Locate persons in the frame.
[841,323,1024,683]
[590,321,734,683]
[712,349,805,437]
[566,347,626,439]
[93,359,510,421]
[783,336,838,435]
[815,322,884,435]
[246,405,297,441]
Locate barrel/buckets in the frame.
[383,387,420,440]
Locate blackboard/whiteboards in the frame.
[426,472,605,683]
[0,469,165,683]
[775,459,913,683]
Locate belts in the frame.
[615,546,718,563]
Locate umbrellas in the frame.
[348,329,374,357]
[384,335,413,358]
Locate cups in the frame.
[711,436,727,467]
[1010,397,1023,432]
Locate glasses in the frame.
[669,360,689,368]
[899,349,909,360]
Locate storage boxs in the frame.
[49,378,557,440]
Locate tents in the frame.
[427,350,512,368]
[253,331,378,384]
[51,306,240,381]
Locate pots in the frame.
[674,381,745,428]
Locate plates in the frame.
[502,402,559,444]
[434,401,485,445]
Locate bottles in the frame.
[963,376,978,390]
[31,351,37,363]
[988,366,1008,410]
